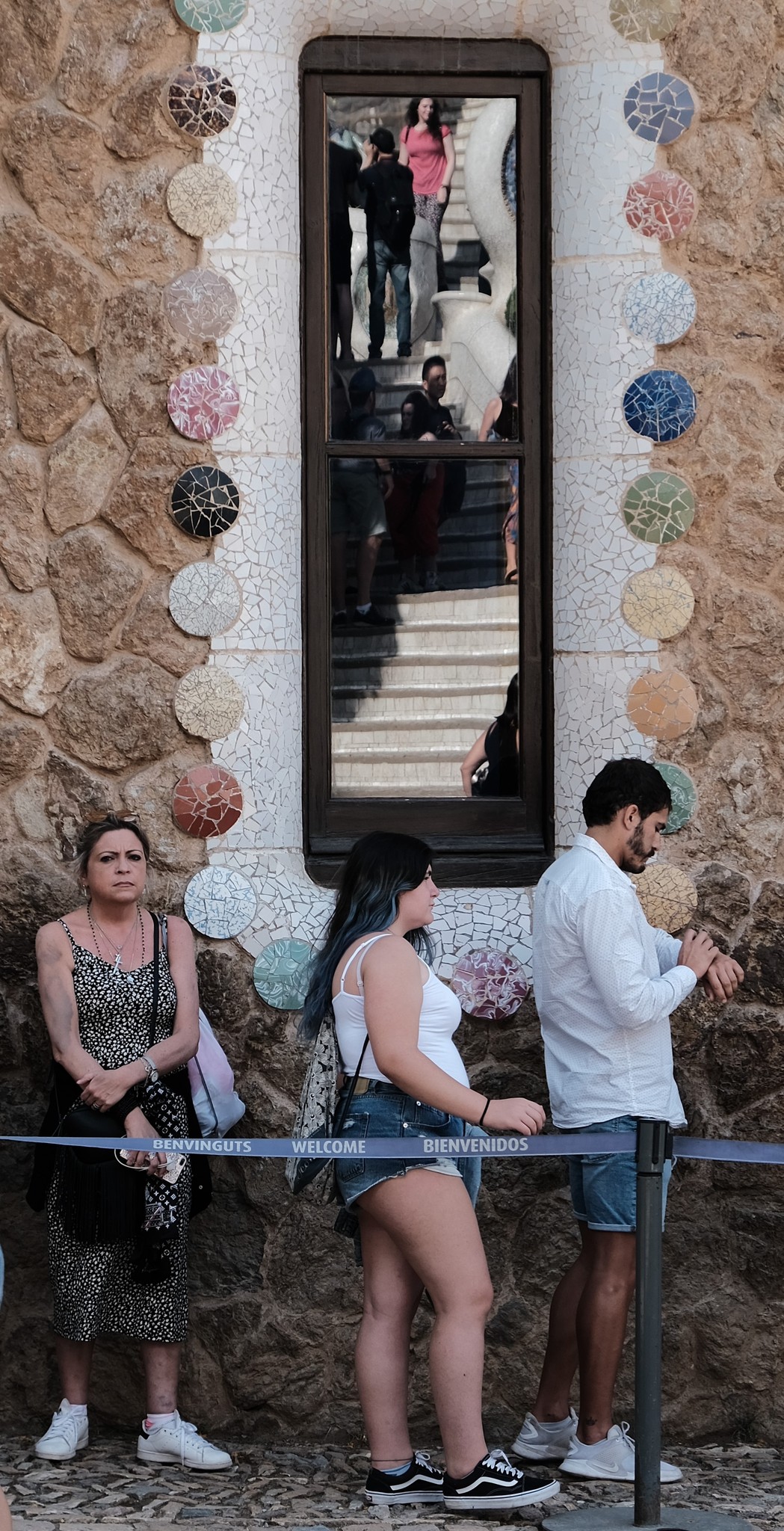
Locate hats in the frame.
[369,127,396,153]
[348,366,384,393]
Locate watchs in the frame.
[141,1055,160,1084]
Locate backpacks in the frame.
[371,164,417,241]
[283,1004,370,1208]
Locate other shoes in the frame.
[352,604,396,627]
[333,612,355,637]
[426,570,441,591]
[389,575,423,593]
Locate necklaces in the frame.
[87,896,145,983]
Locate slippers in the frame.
[505,569,518,585]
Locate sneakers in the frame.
[136,1409,231,1470]
[558,1422,683,1484]
[365,1450,444,1504]
[510,1407,579,1459]
[35,1399,88,1460]
[442,1449,560,1510]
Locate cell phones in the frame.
[120,1149,187,1184]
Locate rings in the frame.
[93,1102,102,1109]
[147,1151,156,1160]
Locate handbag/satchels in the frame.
[187,1008,247,1141]
[66,1106,135,1241]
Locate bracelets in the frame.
[440,184,451,191]
[477,1098,490,1128]
[134,1057,152,1097]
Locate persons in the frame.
[305,826,567,1511]
[29,816,238,1470]
[459,675,523,801]
[391,97,454,290]
[327,369,400,630]
[322,119,362,367]
[514,754,747,1483]
[353,128,418,357]
[477,352,520,585]
[409,354,467,531]
[392,390,445,593]
